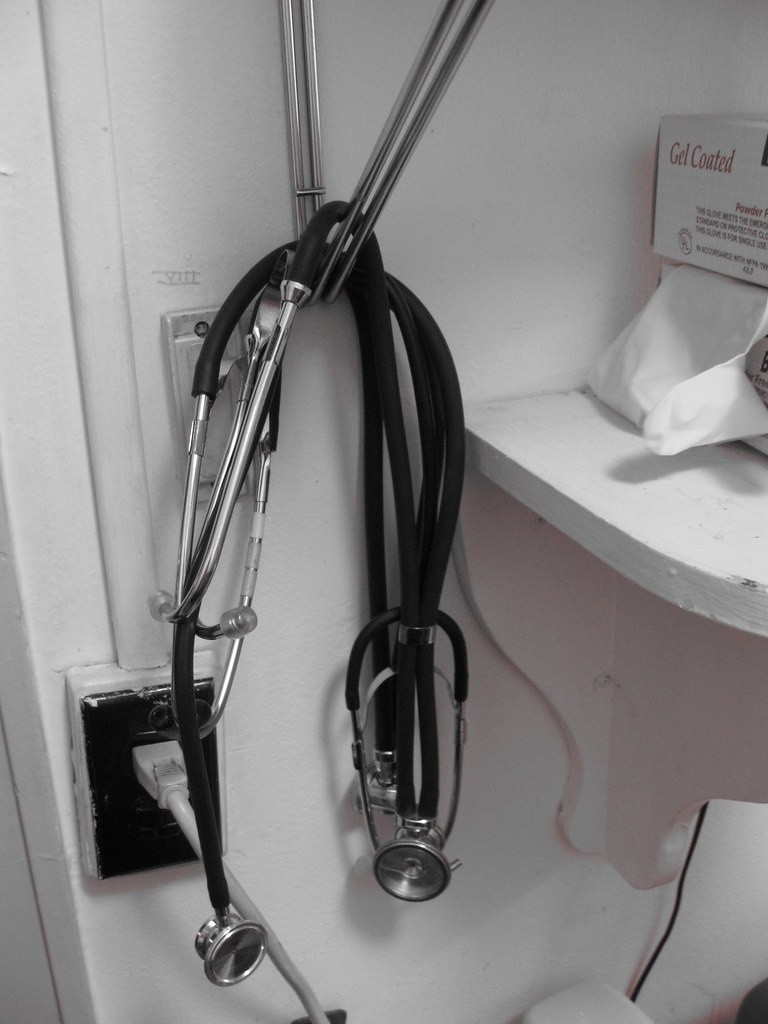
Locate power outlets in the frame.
[65,650,226,881]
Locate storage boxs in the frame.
[657,265,768,456]
[651,114,768,288]
